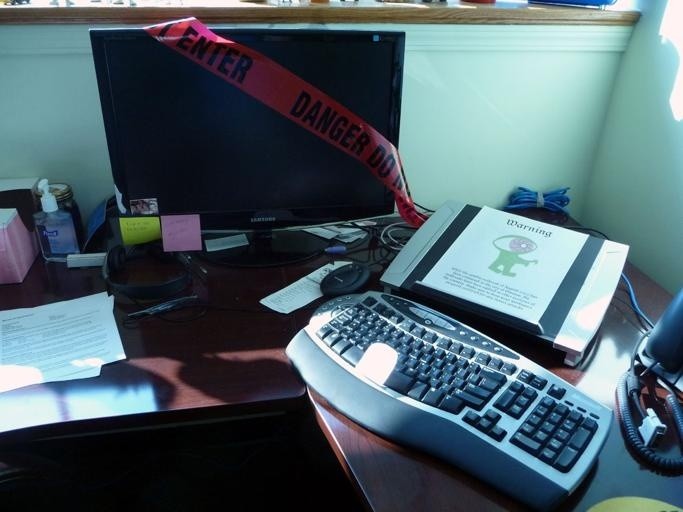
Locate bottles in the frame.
[33,183,84,247]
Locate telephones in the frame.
[636,288,683,393]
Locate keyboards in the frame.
[283,291,616,510]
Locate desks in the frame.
[0,202,682,511]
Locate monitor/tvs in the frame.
[88,27,406,269]
